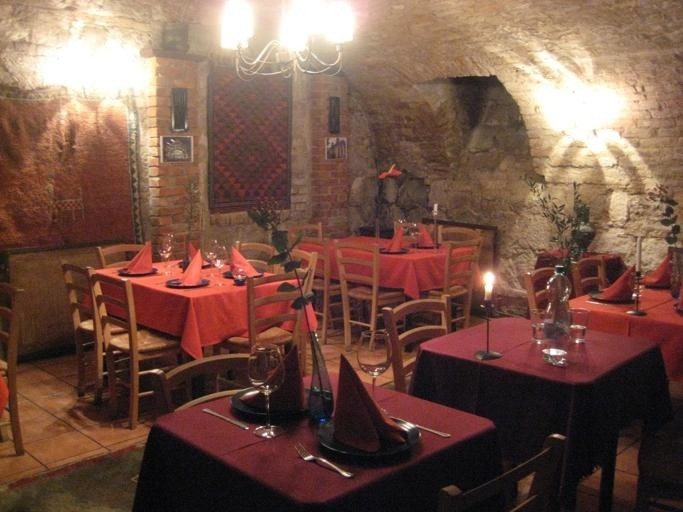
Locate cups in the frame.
[232,265,248,285]
[568,307,591,345]
[631,275,645,297]
[528,305,545,345]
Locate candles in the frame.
[635,235,643,269]
[433,202,438,214]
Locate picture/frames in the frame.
[159,135,193,164]
[325,138,347,161]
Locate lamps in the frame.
[219,0,356,81]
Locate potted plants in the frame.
[521,172,576,338]
[647,182,682,295]
[571,183,596,251]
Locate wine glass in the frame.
[354,329,395,416]
[206,239,229,287]
[156,232,177,274]
[246,341,289,442]
[391,214,425,245]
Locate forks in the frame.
[294,441,357,479]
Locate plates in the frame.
[378,248,409,254]
[163,277,211,289]
[315,416,420,463]
[177,259,208,269]
[585,289,637,304]
[223,268,265,278]
[671,302,682,315]
[414,241,442,249]
[116,266,157,277]
[230,385,317,420]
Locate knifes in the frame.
[201,407,248,430]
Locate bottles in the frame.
[542,264,572,370]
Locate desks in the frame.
[292,233,479,357]
[527,251,623,322]
[85,258,319,398]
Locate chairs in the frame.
[333,239,405,351]
[524,265,556,320]
[286,222,324,248]
[0,282,25,454]
[392,222,435,239]
[158,353,257,413]
[87,266,191,430]
[436,225,482,244]
[58,259,130,411]
[638,447,682,511]
[233,241,275,273]
[266,248,317,295]
[382,294,453,392]
[295,241,351,344]
[570,254,606,294]
[212,269,317,377]
[422,240,482,328]
[97,241,152,268]
[441,432,568,511]
